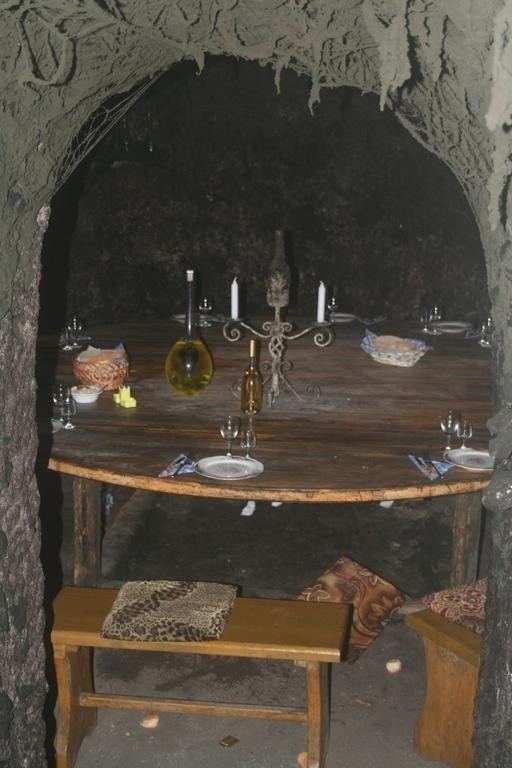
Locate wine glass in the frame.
[419,312,430,333]
[218,416,239,456]
[241,431,256,457]
[67,315,87,348]
[430,307,442,335]
[61,400,77,429]
[51,384,70,406]
[441,410,460,450]
[480,318,494,347]
[455,421,473,450]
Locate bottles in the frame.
[266,230,291,308]
[163,270,214,397]
[241,339,263,416]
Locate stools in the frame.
[404,608,482,767]
[47,587,353,768]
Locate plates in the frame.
[194,455,264,481]
[329,312,357,323]
[431,321,474,334]
[50,418,63,434]
[443,448,494,473]
[169,314,212,324]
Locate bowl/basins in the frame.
[70,385,103,403]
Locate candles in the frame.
[230,276,239,320]
[316,279,326,323]
